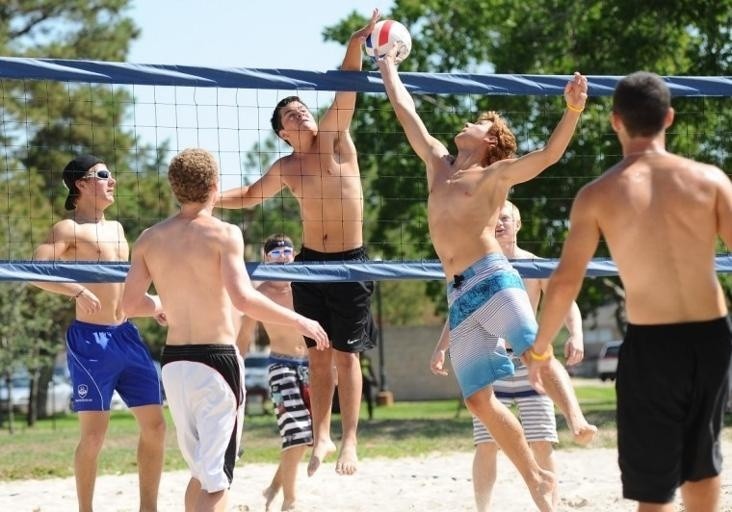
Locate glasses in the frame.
[81,170,112,180]
[268,249,293,257]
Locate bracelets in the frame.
[566,103,584,113]
[531,344,553,361]
[75,287,86,298]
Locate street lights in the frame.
[370,252,396,406]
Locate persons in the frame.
[373,41,596,512]
[528,71,731,511]
[429,199,584,512]
[236,233,337,512]
[209,9,384,474]
[30,154,169,512]
[123,148,329,511]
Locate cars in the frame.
[595,336,625,383]
[0,349,283,417]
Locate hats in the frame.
[62,154,105,211]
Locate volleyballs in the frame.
[365,19,412,63]
